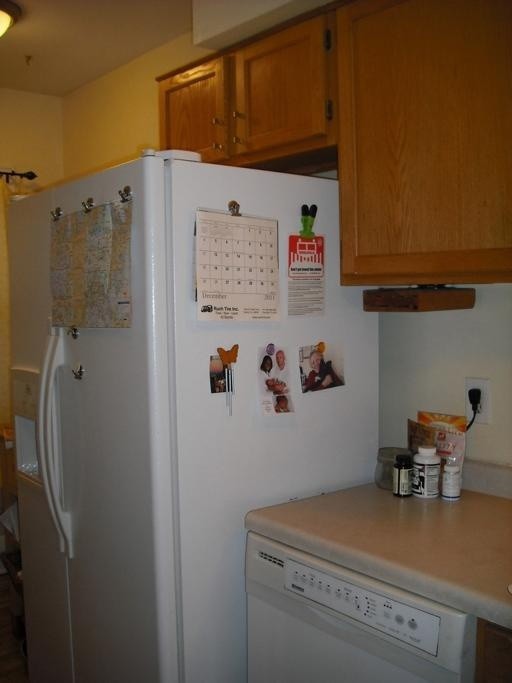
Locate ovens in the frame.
[236,524,468,683]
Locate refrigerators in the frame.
[8,150,379,682]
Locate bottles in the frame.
[444,447,463,502]
[392,451,414,497]
[413,443,443,502]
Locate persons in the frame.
[271,349,289,384]
[274,395,288,412]
[259,354,272,392]
[304,348,334,391]
[266,378,286,391]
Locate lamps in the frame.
[0,0,24,38]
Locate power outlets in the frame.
[463,375,493,426]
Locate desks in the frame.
[242,480,511,683]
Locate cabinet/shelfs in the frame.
[332,0,511,313]
[154,0,338,176]
